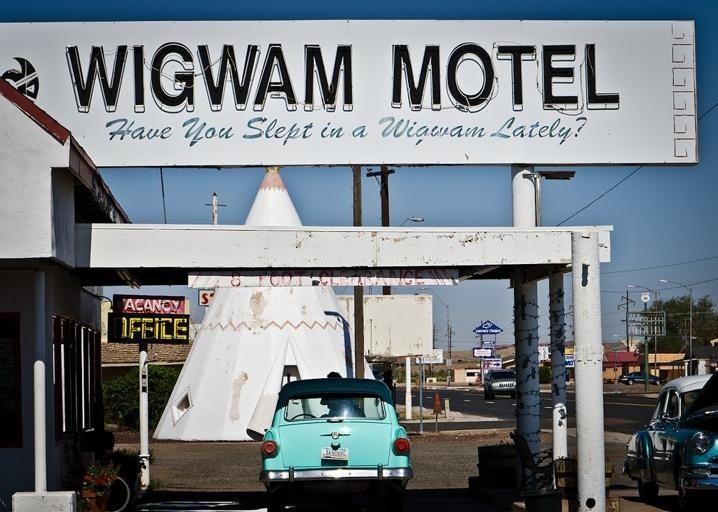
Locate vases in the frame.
[81,489,110,512]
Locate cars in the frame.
[259,377,413,505]
[618,372,664,385]
[622,369,718,512]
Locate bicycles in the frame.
[73,451,131,512]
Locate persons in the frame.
[321,372,353,414]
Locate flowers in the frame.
[78,461,119,496]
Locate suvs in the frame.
[484,369,516,400]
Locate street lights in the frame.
[658,279,692,376]
[419,287,450,353]
[369,216,425,374]
[626,284,657,377]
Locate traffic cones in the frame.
[431,390,443,415]
